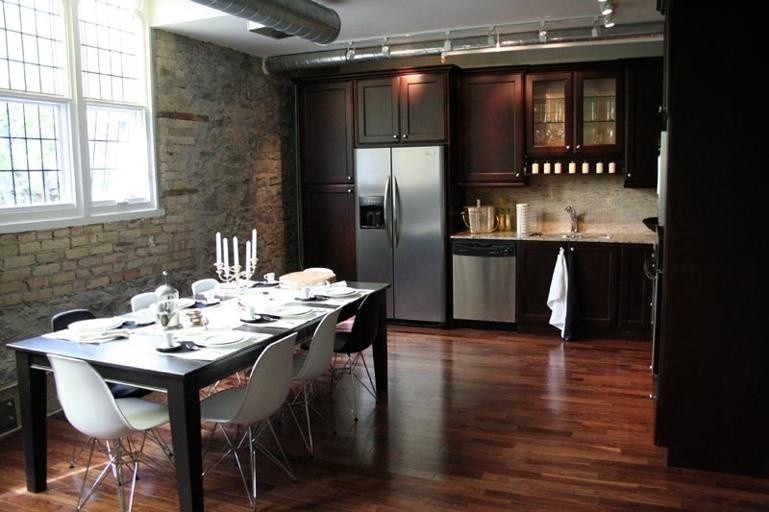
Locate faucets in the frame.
[565,206,578,233]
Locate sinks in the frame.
[530,232,612,242]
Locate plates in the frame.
[64,283,362,350]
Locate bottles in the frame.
[531,100,615,146]
[495,211,512,231]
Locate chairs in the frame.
[45,350,178,511]
[50,307,154,471]
[302,289,382,427]
[129,276,220,314]
[199,330,301,510]
[290,300,350,457]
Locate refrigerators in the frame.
[352,143,449,326]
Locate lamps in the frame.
[337,0,615,61]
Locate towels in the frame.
[546,248,576,341]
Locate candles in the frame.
[233,236,239,265]
[215,232,221,263]
[252,229,257,258]
[245,240,252,276]
[223,237,228,267]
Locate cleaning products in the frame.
[498,207,512,231]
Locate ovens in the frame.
[453,240,519,324]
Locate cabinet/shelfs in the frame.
[294,71,356,282]
[617,243,653,341]
[522,59,624,159]
[354,64,460,148]
[623,57,664,189]
[459,63,524,188]
[517,241,619,341]
